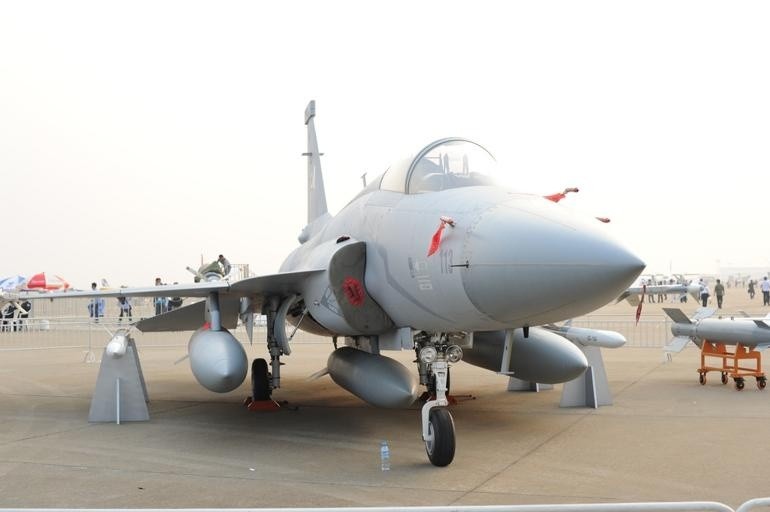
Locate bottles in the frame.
[380,440,391,472]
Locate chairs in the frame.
[389,158,457,195]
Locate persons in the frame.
[89,282,99,321]
[218,255,233,277]
[152,277,183,316]
[17,300,31,331]
[117,285,132,321]
[2,300,20,331]
[646,274,770,309]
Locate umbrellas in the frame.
[18,272,69,293]
[0,277,25,291]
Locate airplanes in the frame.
[0,99,705,467]
